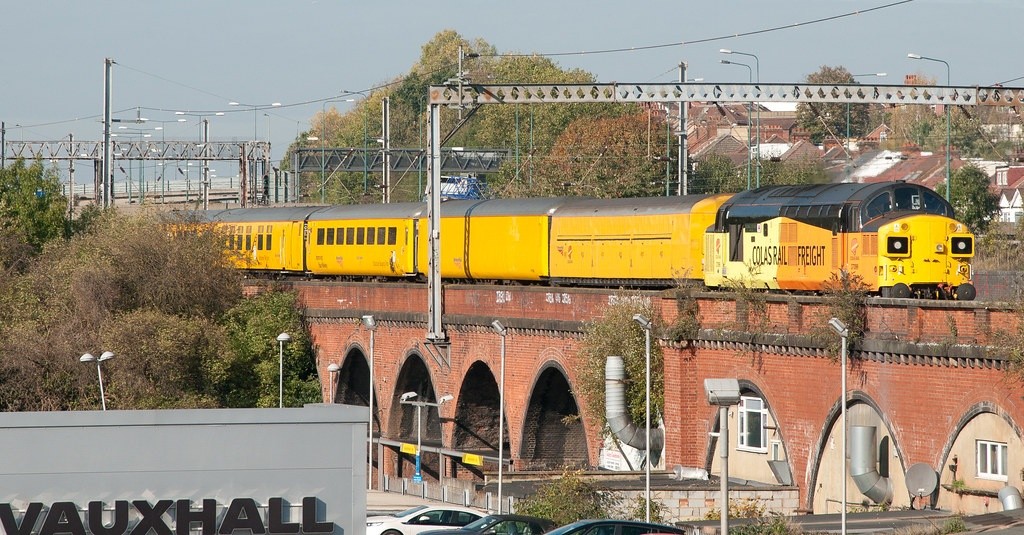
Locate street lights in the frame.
[491,318,508,516]
[845,72,889,183]
[907,53,952,204]
[631,313,651,524]
[362,313,376,490]
[228,101,282,205]
[719,48,760,189]
[718,59,752,192]
[276,332,292,408]
[174,111,226,201]
[338,89,367,195]
[487,75,534,198]
[112,118,187,204]
[79,350,115,411]
[827,316,851,535]
[665,78,705,198]
[321,98,355,208]
[327,363,341,403]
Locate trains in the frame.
[156,178,977,303]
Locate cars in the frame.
[415,514,559,535]
[366,505,502,535]
[541,517,686,535]
